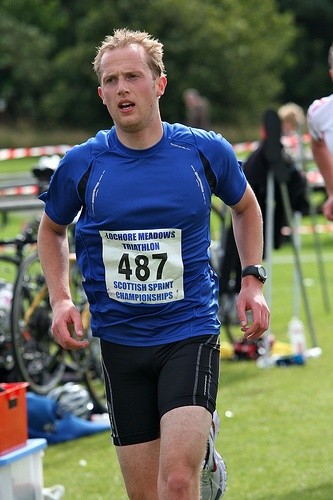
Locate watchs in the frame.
[241,264,267,284]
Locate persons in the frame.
[37,26,270,500]
[181,44,333,296]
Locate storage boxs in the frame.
[1,382,49,499]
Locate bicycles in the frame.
[1,227,108,413]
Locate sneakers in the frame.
[199,409,228,499]
